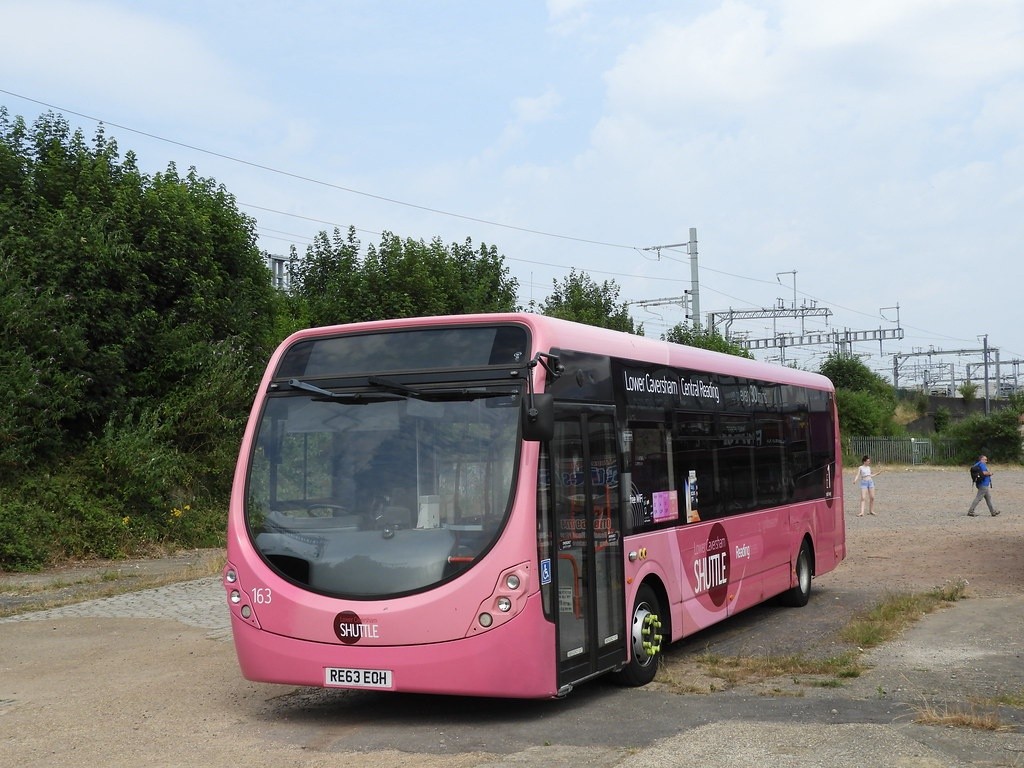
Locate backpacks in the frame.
[971,463,985,483]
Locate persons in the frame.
[853,456,881,517]
[967,455,1000,516]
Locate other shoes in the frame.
[967,513,979,517]
[992,511,1000,517]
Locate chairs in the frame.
[553,419,813,518]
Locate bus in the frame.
[220,313,847,703]
